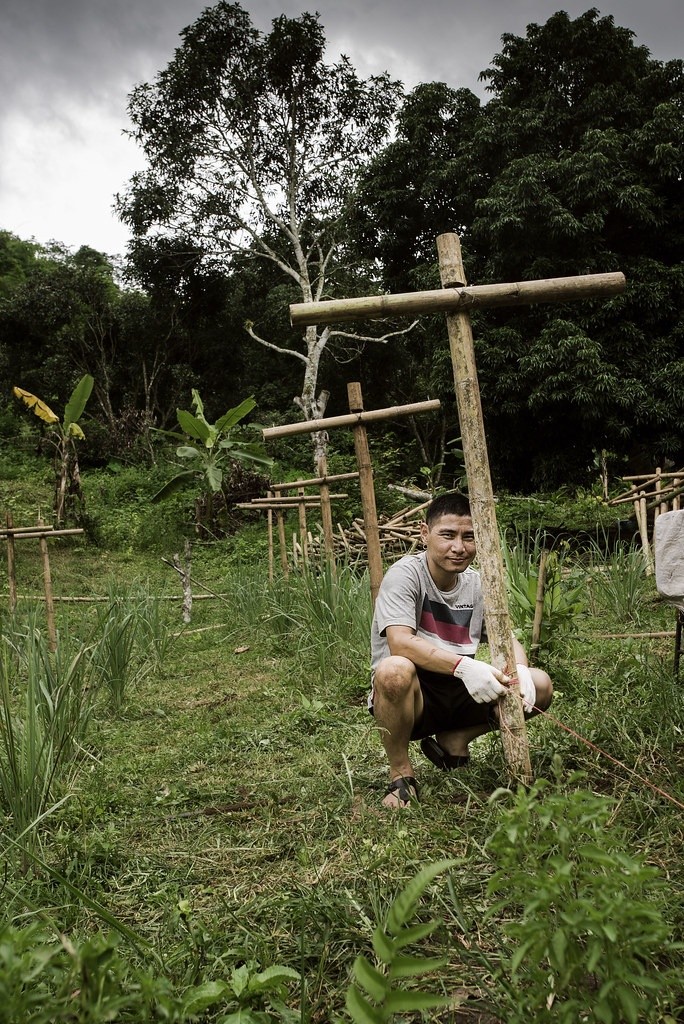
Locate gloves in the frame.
[453,655,510,704]
[516,662,536,714]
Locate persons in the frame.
[368,494,554,810]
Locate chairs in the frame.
[653,509,684,678]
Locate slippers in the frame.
[421,738,468,771]
[386,774,420,806]
